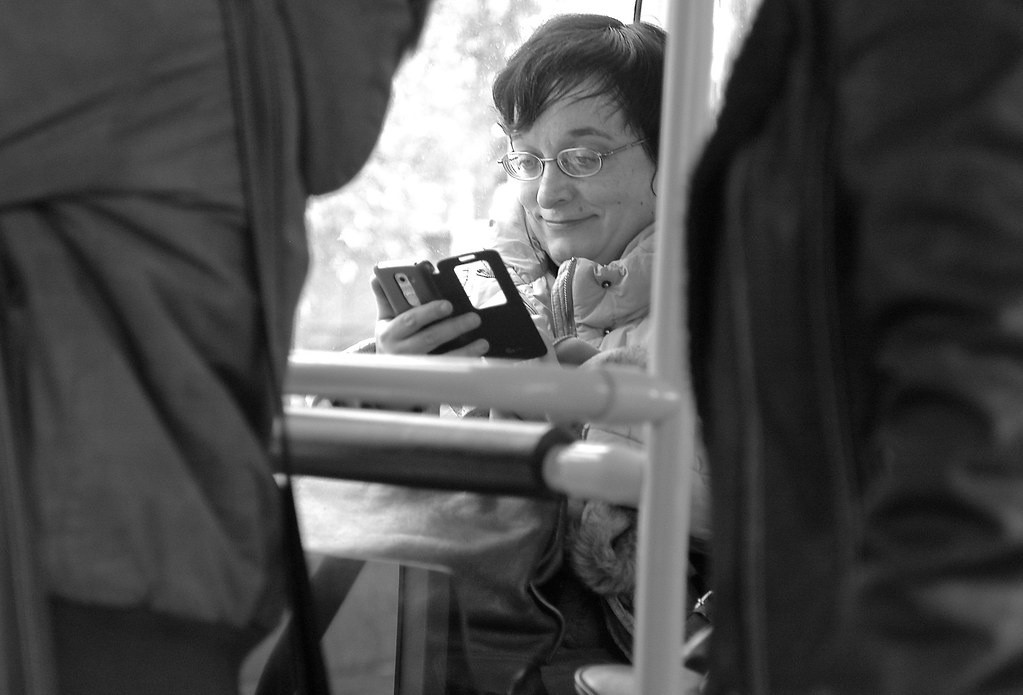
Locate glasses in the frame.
[498,138,651,181]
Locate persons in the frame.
[0,1,433,695]
[684,0,1023,695]
[305,12,712,695]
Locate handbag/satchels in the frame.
[291,466,628,695]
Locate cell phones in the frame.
[374,259,461,357]
